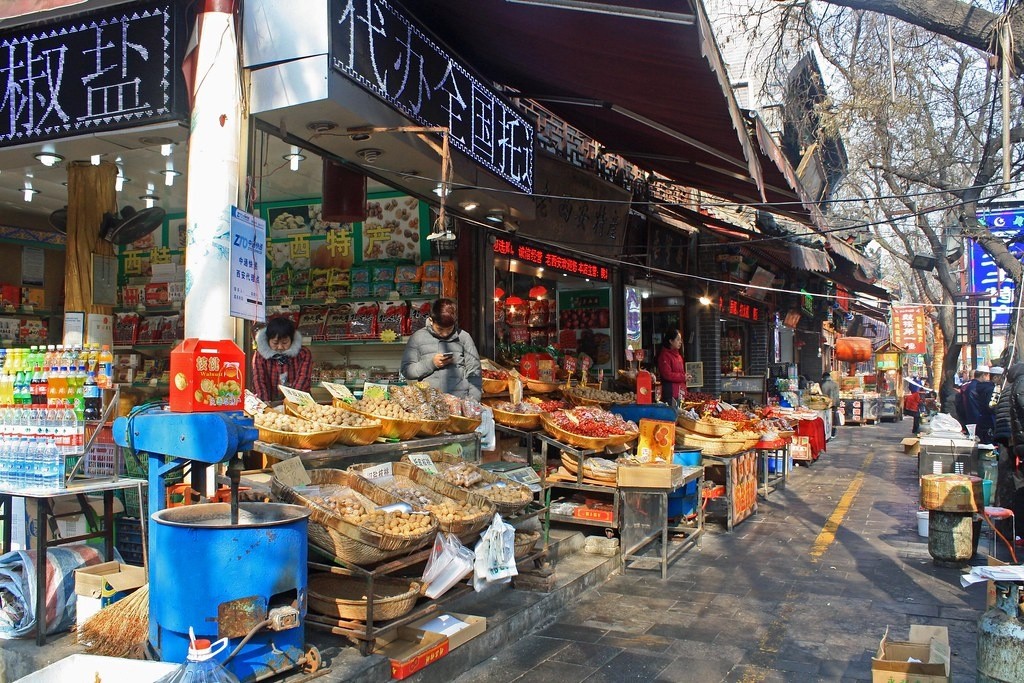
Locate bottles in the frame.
[0,437,65,491]
[153,637,240,683]
[0,404,83,455]
[774,376,798,392]
[65,455,85,474]
[0,366,103,420]
[0,343,114,389]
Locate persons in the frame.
[975,367,1005,444]
[995,361,1024,549]
[906,391,937,434]
[400,298,483,403]
[954,365,990,436]
[658,328,692,403]
[909,374,925,412]
[820,372,840,438]
[252,318,312,406]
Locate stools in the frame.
[983,506,1017,559]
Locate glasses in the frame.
[675,339,683,341]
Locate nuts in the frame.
[250,395,420,432]
[567,386,637,402]
[299,462,530,537]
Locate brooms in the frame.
[68,582,150,658]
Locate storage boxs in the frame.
[702,484,724,498]
[900,437,919,455]
[11,497,26,551]
[984,552,1024,612]
[71,560,150,646]
[637,372,652,405]
[784,437,792,444]
[121,447,184,479]
[636,416,676,465]
[84,421,115,442]
[868,621,955,683]
[126,478,183,518]
[574,501,621,523]
[26,496,54,550]
[361,623,451,679]
[759,453,795,474]
[406,608,489,652]
[66,455,84,473]
[53,496,125,547]
[519,353,556,381]
[87,488,125,514]
[116,516,148,566]
[756,438,785,449]
[617,461,685,490]
[81,442,126,478]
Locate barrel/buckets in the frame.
[917,511,929,537]
[780,396,793,407]
[983,480,993,506]
[762,456,793,472]
[668,444,704,518]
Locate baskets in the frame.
[778,430,795,438]
[479,357,641,449]
[254,397,534,564]
[307,573,421,621]
[675,415,763,456]
[560,444,630,483]
[513,528,541,558]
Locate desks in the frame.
[792,417,826,468]
[814,408,833,441]
[0,478,147,648]
[756,445,788,501]
[621,466,705,580]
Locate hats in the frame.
[989,366,1004,374]
[976,366,989,372]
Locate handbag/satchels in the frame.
[1011,443,1024,476]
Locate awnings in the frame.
[391,1,885,282]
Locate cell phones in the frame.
[443,353,453,359]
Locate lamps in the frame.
[494,286,505,300]
[504,296,522,314]
[528,276,547,301]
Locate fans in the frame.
[96,205,166,246]
[48,205,68,235]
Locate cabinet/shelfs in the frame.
[229,431,555,652]
[493,421,622,536]
[261,292,440,389]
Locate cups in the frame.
[118,398,131,416]
